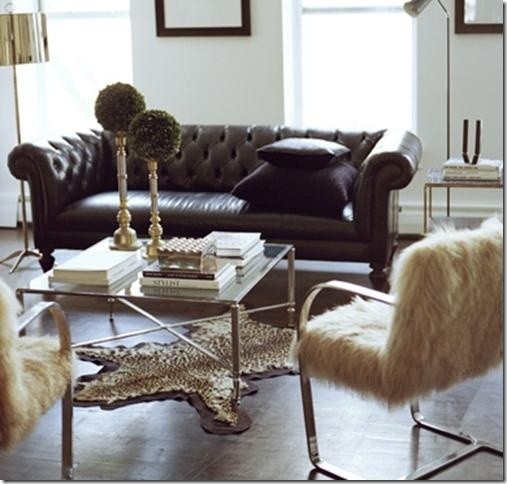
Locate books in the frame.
[142,286,237,299]
[140,258,238,289]
[440,158,502,181]
[200,231,266,269]
[47,236,146,286]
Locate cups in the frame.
[427,166,442,183]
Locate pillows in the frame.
[228,159,359,217]
[253,136,354,168]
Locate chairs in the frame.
[294,217,507,484]
[0,274,76,484]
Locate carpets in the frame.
[69,300,300,436]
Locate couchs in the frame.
[5,119,423,294]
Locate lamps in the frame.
[1,11,50,275]
[402,0,450,234]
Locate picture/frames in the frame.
[152,0,253,39]
[454,0,504,34]
